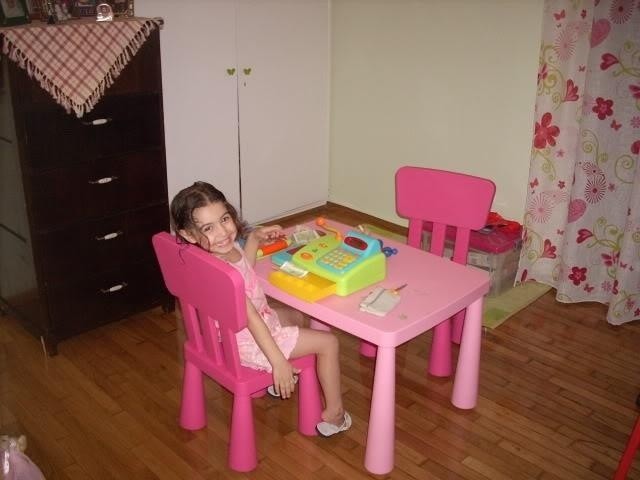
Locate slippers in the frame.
[316,411,352,437]
[268,375,299,396]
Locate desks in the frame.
[254,219,490,474]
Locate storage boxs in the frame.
[421,230,524,298]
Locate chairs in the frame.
[153,231,324,472]
[360,166,496,377]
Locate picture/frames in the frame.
[0,0,136,27]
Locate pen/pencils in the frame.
[395,284,409,291]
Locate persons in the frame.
[169,180,353,439]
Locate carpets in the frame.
[357,223,553,330]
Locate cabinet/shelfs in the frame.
[135,1,330,227]
[1,19,179,358]
